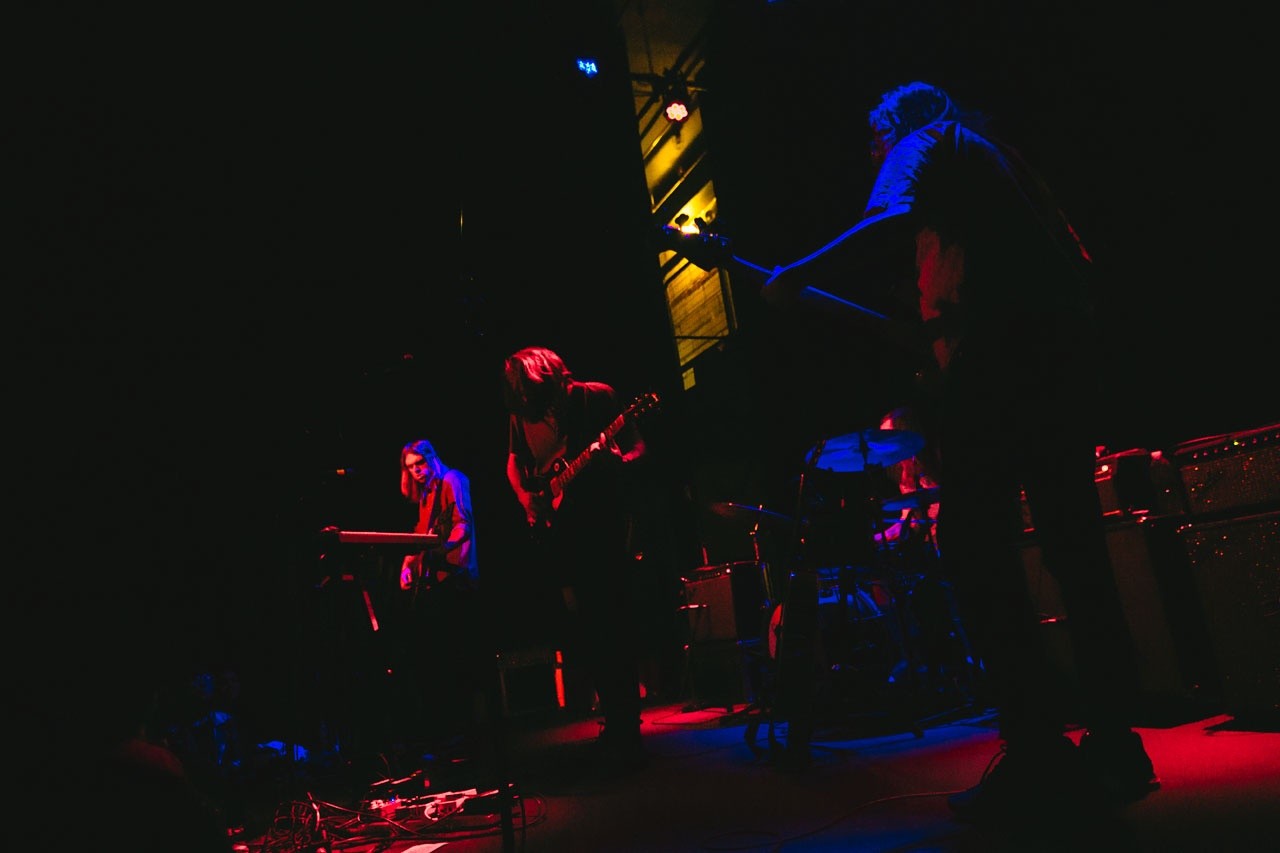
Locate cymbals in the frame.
[803,430,928,473]
[881,487,942,512]
[710,500,794,528]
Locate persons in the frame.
[400,439,471,742]
[501,345,646,777]
[765,82,1158,813]
[872,409,941,685]
[89,654,260,853]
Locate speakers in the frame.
[1017,423,1279,732]
[679,561,771,704]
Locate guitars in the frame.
[410,500,457,617]
[512,389,666,546]
[654,219,967,402]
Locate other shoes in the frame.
[1043,727,1154,816]
[946,741,1070,827]
[583,730,648,775]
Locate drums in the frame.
[888,574,968,668]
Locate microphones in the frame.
[329,469,353,475]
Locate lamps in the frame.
[662,86,692,123]
